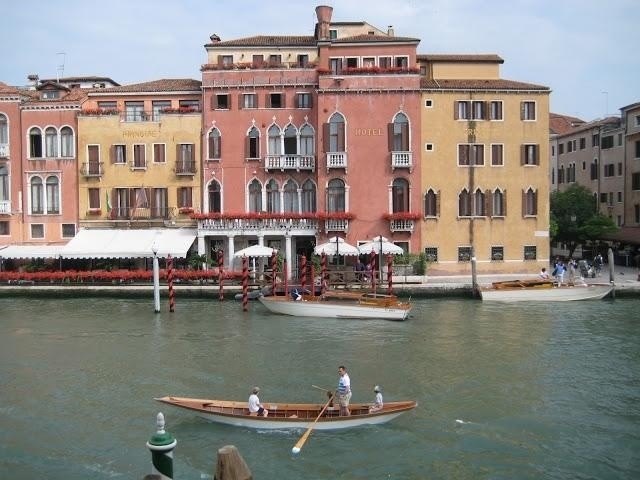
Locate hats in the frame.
[374,386,380,391]
[253,387,262,392]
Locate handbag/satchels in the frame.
[552,269,558,276]
[574,263,578,269]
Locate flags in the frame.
[106,192,112,212]
[129,187,147,219]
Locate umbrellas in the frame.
[357,236,405,283]
[234,244,279,283]
[313,236,360,271]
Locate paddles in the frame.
[312,385,337,397]
[292,390,337,454]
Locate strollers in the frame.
[579,260,595,277]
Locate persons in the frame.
[335,366,352,416]
[352,260,366,284]
[540,253,608,288]
[291,288,312,301]
[368,385,384,413]
[319,275,329,299]
[248,386,269,417]
[365,262,373,280]
[214,444,253,480]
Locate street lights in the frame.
[151,241,161,312]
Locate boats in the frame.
[255,279,414,322]
[475,279,616,302]
[151,395,421,435]
